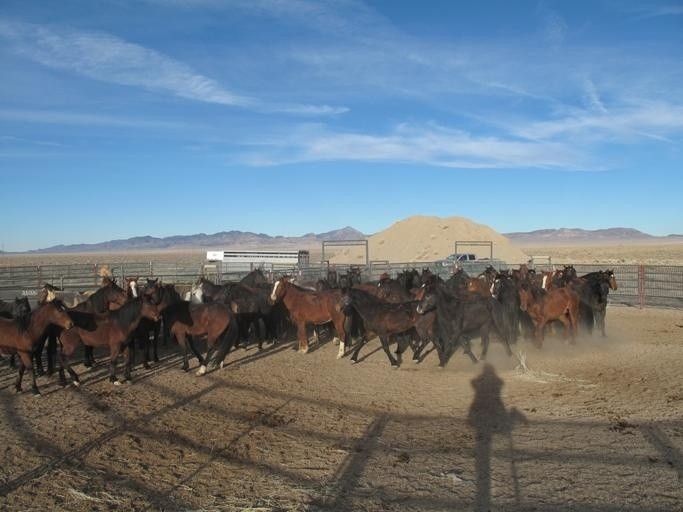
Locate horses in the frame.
[0,263,619,398]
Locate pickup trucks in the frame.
[444,252,489,260]
